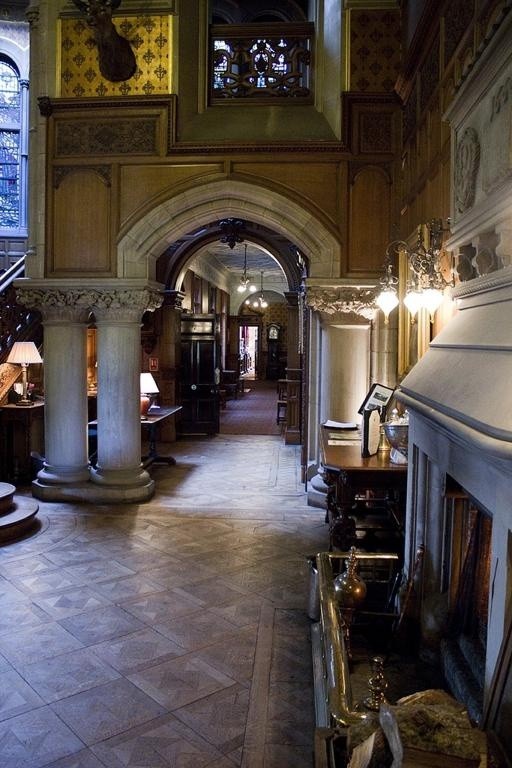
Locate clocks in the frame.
[265,324,282,380]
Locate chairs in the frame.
[279,386,287,400]
[276,400,287,425]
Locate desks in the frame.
[219,390,226,410]
[320,420,408,552]
[277,379,286,393]
[88,405,185,470]
[222,380,244,400]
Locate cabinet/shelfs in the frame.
[223,371,238,384]
[0,400,45,480]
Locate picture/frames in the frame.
[397,225,429,383]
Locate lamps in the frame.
[237,243,249,295]
[6,341,43,405]
[140,373,160,421]
[246,271,271,310]
[372,228,441,325]
